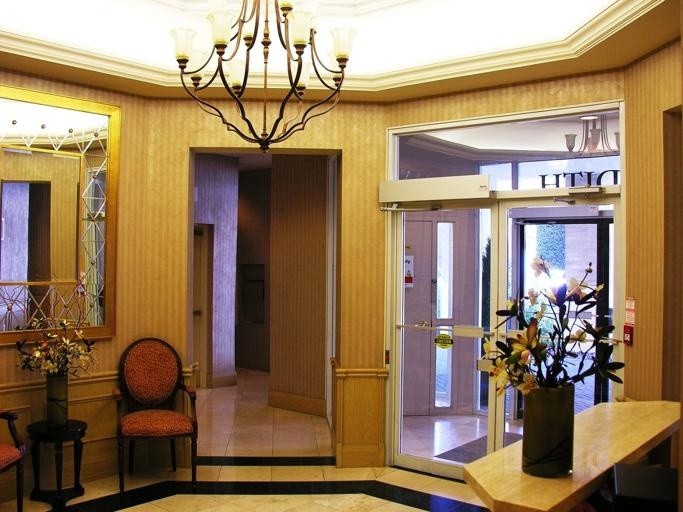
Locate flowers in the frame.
[480,255,626,397]
[15,320,98,377]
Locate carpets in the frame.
[435,432,522,463]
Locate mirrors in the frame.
[0,83,122,345]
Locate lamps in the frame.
[175,0,359,150]
[564,113,619,158]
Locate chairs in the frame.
[112,337,198,493]
[0,410,27,512]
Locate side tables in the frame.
[27,419,87,507]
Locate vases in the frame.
[522,380,575,480]
[47,372,69,423]
